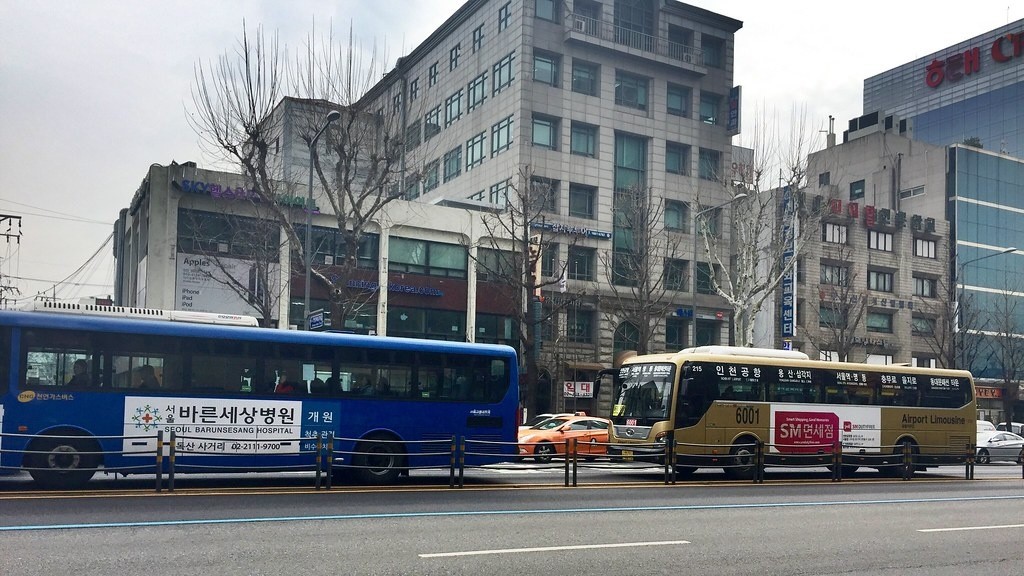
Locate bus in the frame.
[607,345,976,478]
[0,301,520,489]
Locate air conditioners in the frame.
[680,52,696,64]
[573,18,587,33]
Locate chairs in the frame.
[309,377,388,394]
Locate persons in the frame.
[241,368,294,393]
[67,360,88,386]
[138,365,160,387]
[327,369,391,395]
[722,387,739,398]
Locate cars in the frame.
[509,416,612,464]
[976,431,1024,464]
[519,414,577,432]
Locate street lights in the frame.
[691,193,747,348]
[303,111,341,331]
[960,247,1016,371]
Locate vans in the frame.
[996,422,1024,438]
[977,420,996,431]
